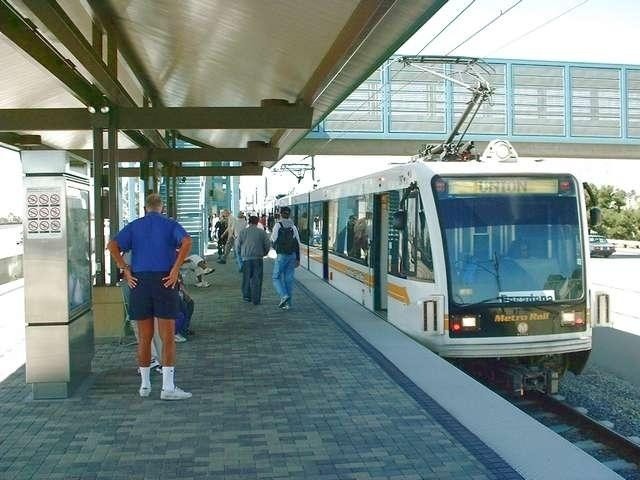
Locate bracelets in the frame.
[121,264,128,272]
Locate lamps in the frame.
[86,92,114,115]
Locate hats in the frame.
[280,207,290,214]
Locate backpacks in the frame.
[273,221,298,255]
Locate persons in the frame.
[271,207,301,310]
[335,212,373,259]
[106,194,192,400]
[208,209,275,264]
[299,213,322,247]
[180,254,215,288]
[174,277,194,343]
[235,216,270,305]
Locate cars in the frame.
[588,234,616,260]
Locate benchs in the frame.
[115,275,140,347]
[178,266,193,280]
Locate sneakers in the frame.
[194,266,216,277]
[160,385,192,400]
[136,356,163,375]
[138,386,152,397]
[174,327,195,343]
[194,279,212,288]
[278,295,292,310]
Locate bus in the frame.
[251,158,591,400]
